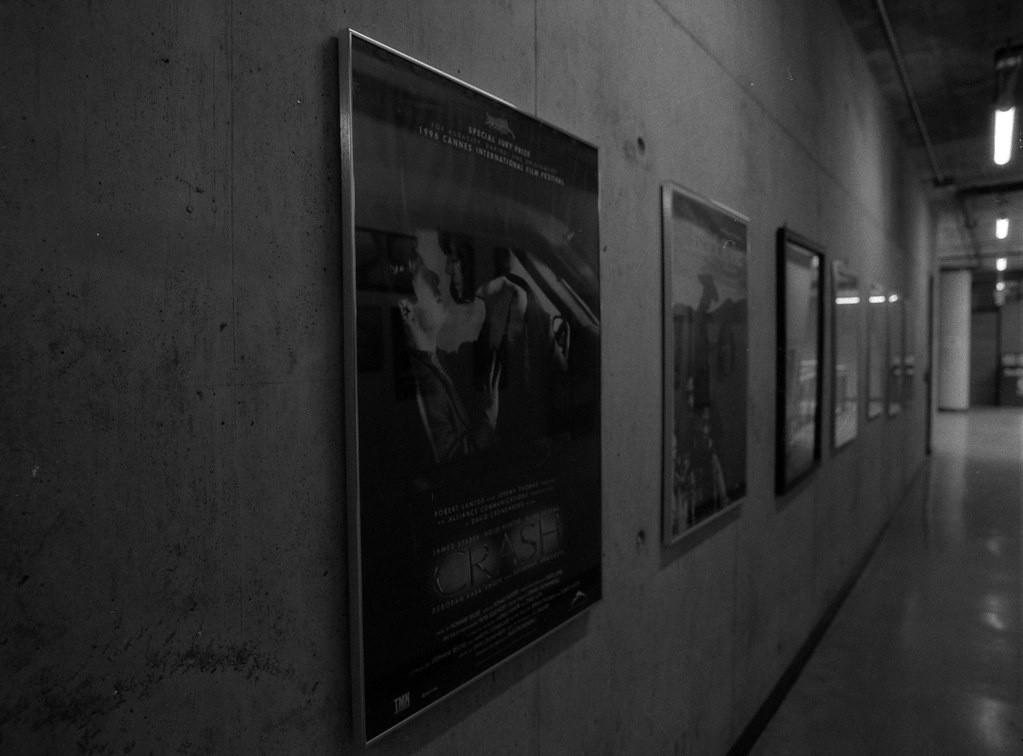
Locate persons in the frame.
[386,225,601,676]
